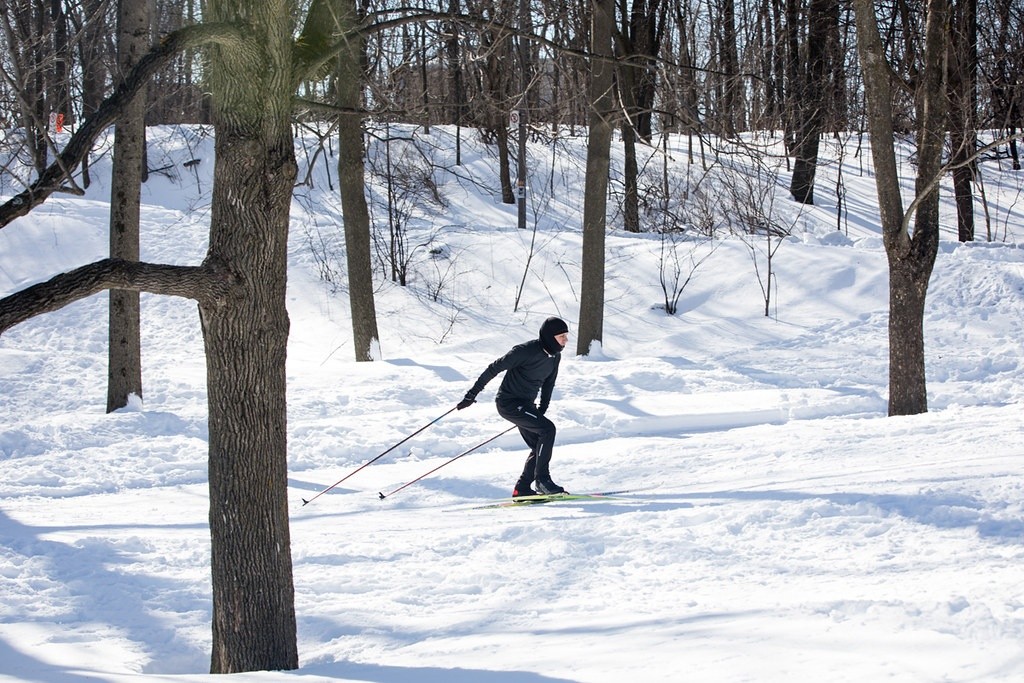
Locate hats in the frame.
[547,317,569,336]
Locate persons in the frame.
[457,317,569,502]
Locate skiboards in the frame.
[443,481,665,513]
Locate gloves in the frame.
[461,390,476,408]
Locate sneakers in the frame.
[512,482,544,502]
[535,477,564,495]
